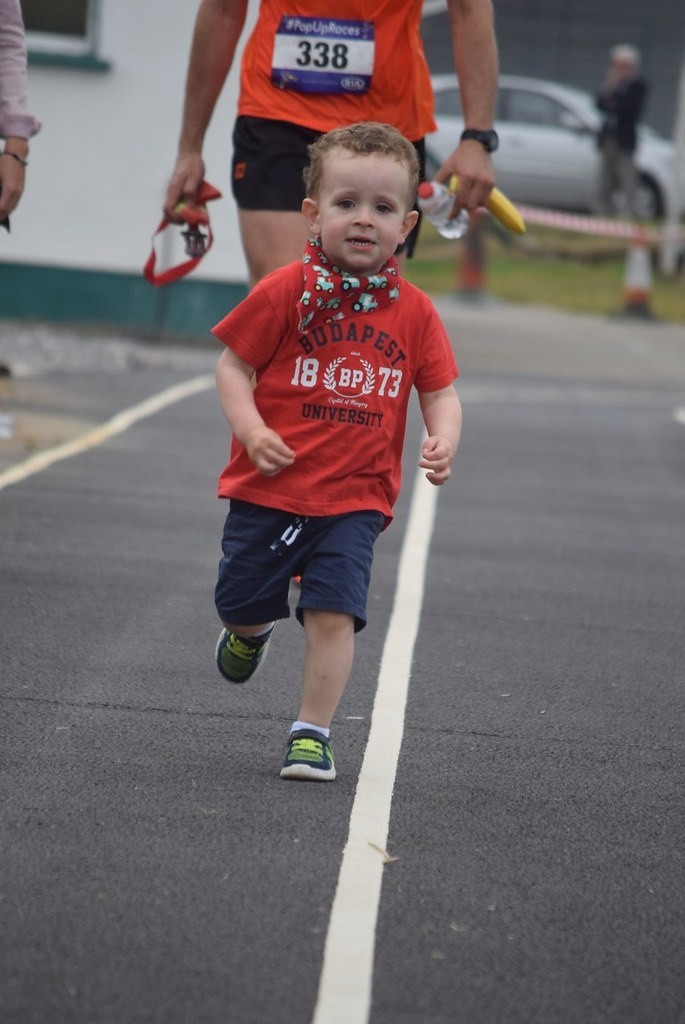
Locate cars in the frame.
[424,72,685,225]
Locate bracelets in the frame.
[4,151,28,166]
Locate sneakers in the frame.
[215,618,281,686]
[281,726,336,784]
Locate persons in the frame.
[598,44,648,221]
[144,0,498,291]
[0,0,41,221]
[210,122,463,781]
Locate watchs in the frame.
[461,128,496,153]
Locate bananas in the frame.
[447,174,526,235]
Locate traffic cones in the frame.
[448,211,488,299]
[616,227,656,319]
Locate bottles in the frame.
[418,180,470,239]
